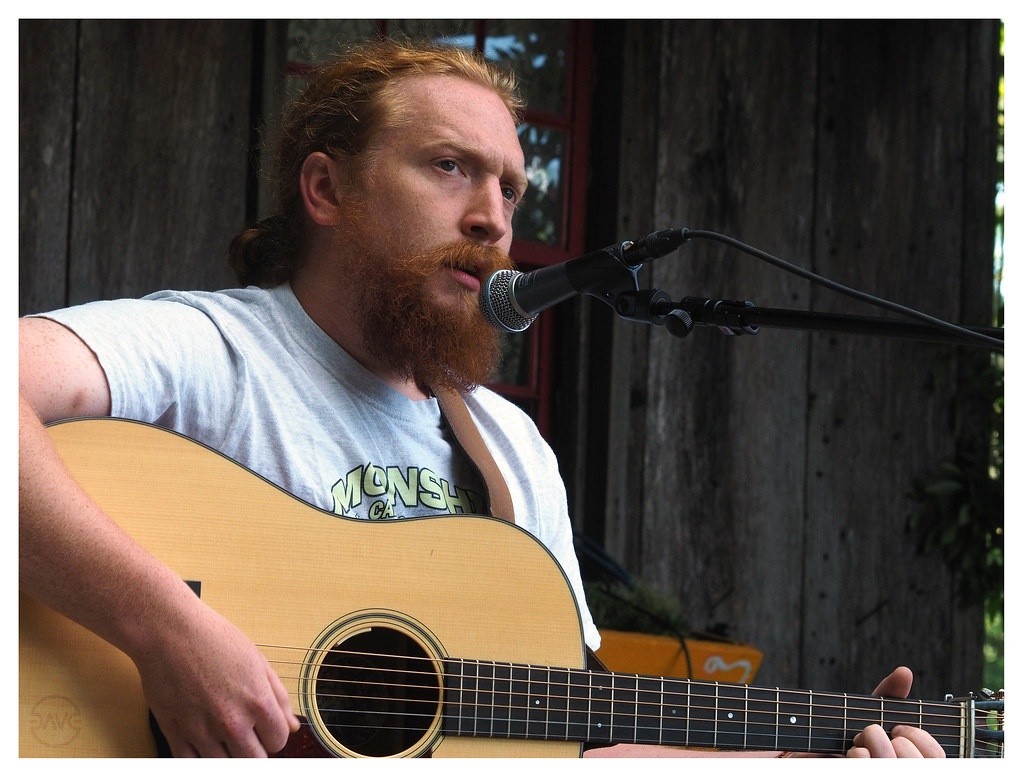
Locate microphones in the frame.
[479,228,686,334]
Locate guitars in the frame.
[18,416,1004,758]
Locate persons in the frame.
[20,44,947,758]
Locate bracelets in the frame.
[777,751,795,758]
